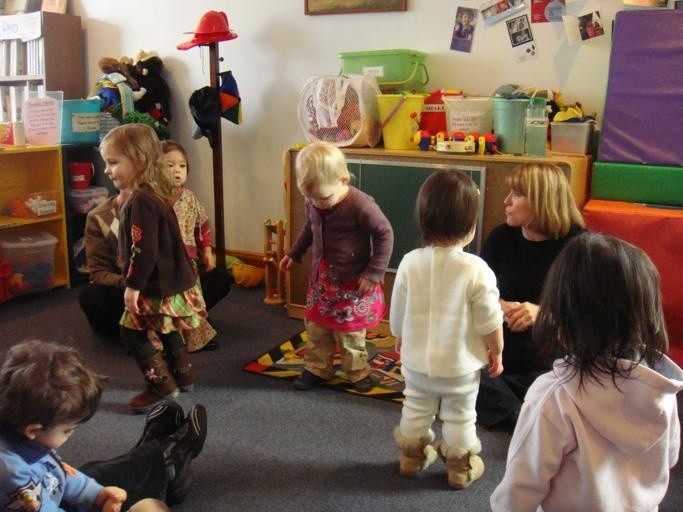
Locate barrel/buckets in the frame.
[377,93,424,149]
[62,99,104,145]
[491,99,529,153]
[68,163,94,189]
[440,95,491,135]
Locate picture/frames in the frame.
[304,0,407,15]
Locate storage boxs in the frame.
[0,227,59,296]
[337,49,430,94]
[550,122,594,154]
[71,186,109,214]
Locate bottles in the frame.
[525,98,549,157]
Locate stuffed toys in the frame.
[83,51,172,124]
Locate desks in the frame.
[283,144,594,337]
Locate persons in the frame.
[388,169,505,488]
[77,181,234,350]
[1,340,171,512]
[157,141,216,354]
[479,162,593,432]
[279,143,394,391]
[453,9,475,43]
[486,232,682,511]
[78,400,209,512]
[96,124,210,414]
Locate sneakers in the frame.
[355,374,372,392]
[293,370,327,391]
[162,404,207,504]
[138,399,184,448]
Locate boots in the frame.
[435,438,485,489]
[130,351,179,414]
[392,425,438,476]
[167,347,194,393]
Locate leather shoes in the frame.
[203,340,218,351]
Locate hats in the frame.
[189,85,219,148]
[220,71,242,124]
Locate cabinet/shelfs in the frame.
[61,132,170,288]
[0,10,86,123]
[0,145,71,302]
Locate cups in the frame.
[13,122,25,145]
